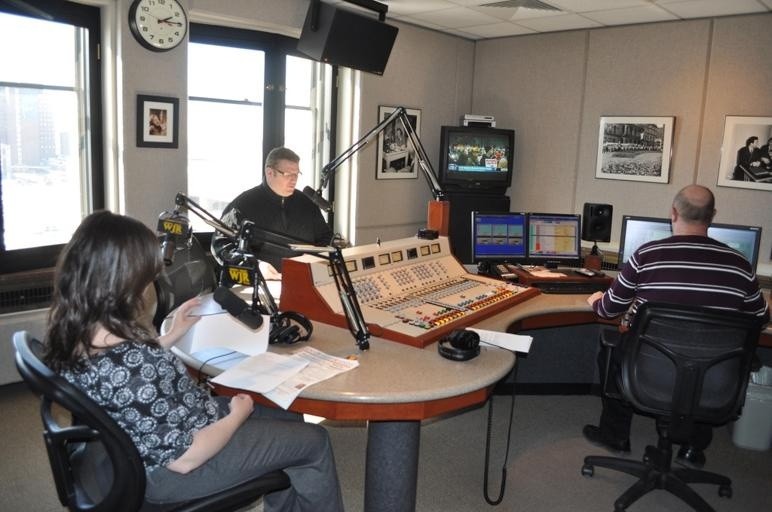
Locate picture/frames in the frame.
[136,94,179,149]
[595,114,676,184]
[375,105,422,180]
[715,114,772,193]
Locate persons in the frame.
[760,137,772,161]
[43,209,346,512]
[586,184,770,468]
[395,127,405,151]
[159,110,167,132]
[449,143,508,171]
[209,147,334,281]
[148,113,162,135]
[731,136,770,179]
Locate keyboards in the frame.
[602,261,617,271]
[531,282,609,294]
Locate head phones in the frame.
[438,327,481,361]
[269,311,313,344]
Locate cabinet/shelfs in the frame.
[446,196,509,256]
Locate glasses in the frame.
[273,168,302,177]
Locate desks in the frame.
[160,265,772,512]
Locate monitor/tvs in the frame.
[525,215,581,269]
[617,215,673,270]
[439,125,515,194]
[470,210,527,275]
[707,223,762,273]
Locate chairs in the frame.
[12,330,292,512]
[580,303,763,512]
[152,234,219,331]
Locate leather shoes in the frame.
[584,424,630,456]
[675,443,709,470]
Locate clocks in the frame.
[129,0,188,51]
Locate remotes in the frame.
[575,267,595,278]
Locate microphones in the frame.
[156,210,193,266]
[302,186,330,211]
[213,286,263,329]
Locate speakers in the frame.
[295,0,399,76]
[582,203,613,242]
